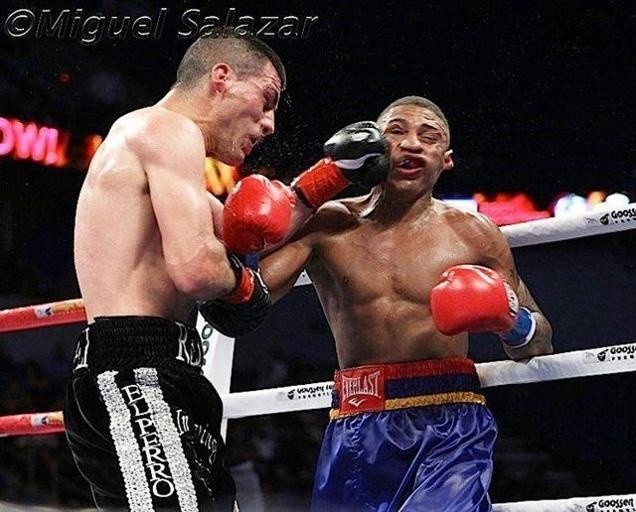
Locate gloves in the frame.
[430,265,537,348]
[222,175,290,268]
[200,254,272,339]
[292,120,390,211]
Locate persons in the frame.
[61,26,394,511]
[218,91,557,512]
